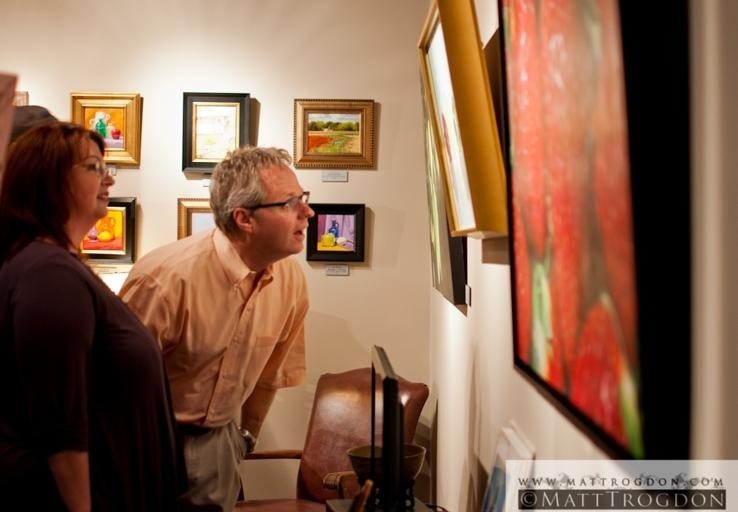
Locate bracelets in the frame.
[234,424,260,455]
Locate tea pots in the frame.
[96,216,117,242]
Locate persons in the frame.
[8,100,64,254]
[117,145,318,511]
[1,119,185,512]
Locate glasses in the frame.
[252,190,309,210]
[84,161,117,176]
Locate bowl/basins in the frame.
[346,442,427,484]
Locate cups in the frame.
[95,112,111,123]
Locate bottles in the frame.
[96,119,106,138]
[328,220,338,238]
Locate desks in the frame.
[325,494,434,512]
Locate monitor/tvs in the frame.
[370,345,405,512]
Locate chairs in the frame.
[223,367,430,512]
